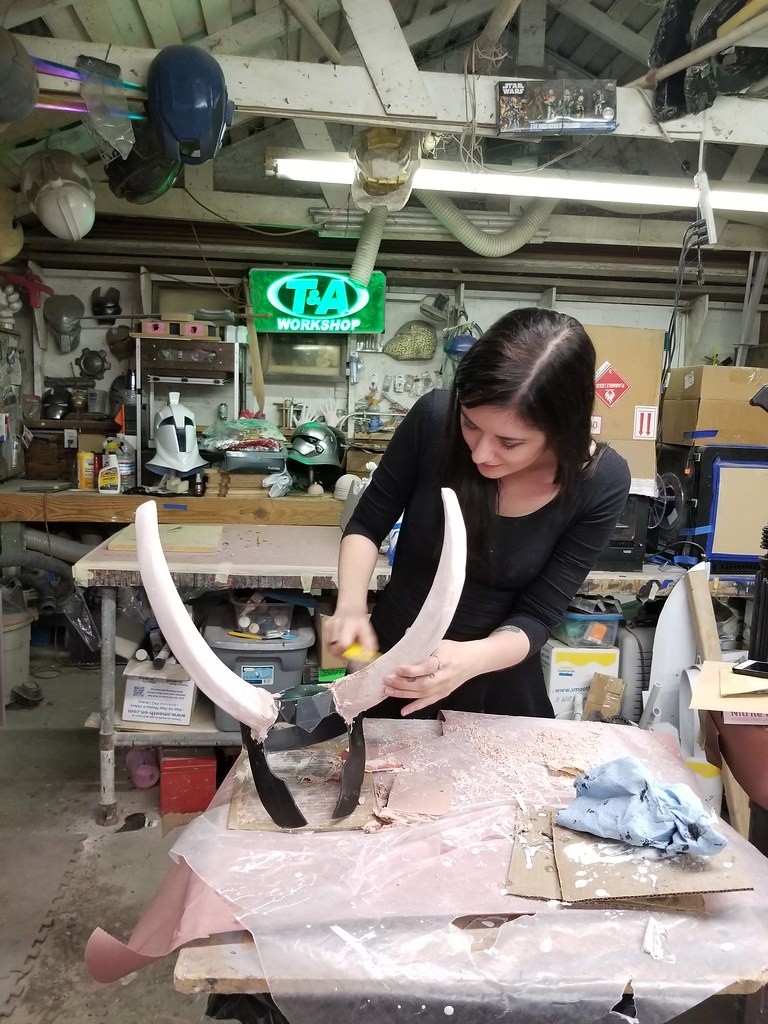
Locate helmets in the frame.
[141,46,235,164]
[288,422,344,468]
[1,188,23,267]
[101,123,182,207]
[1,26,39,124]
[144,391,211,479]
[20,148,96,240]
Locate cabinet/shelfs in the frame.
[130,330,246,488]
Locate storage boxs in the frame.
[315,601,375,669]
[539,638,621,720]
[581,324,665,499]
[116,606,199,726]
[552,611,625,648]
[203,606,315,733]
[660,364,768,446]
[2,616,36,706]
[346,449,384,478]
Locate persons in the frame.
[323,306,632,718]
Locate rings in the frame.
[432,654,443,671]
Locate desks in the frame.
[172,715,768,1024]
[71,523,758,829]
[0,479,344,526]
[22,418,122,485]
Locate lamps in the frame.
[262,147,768,215]
